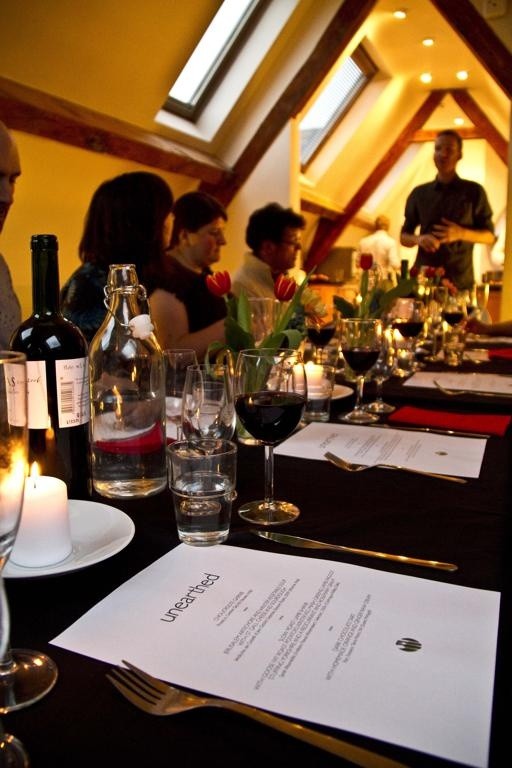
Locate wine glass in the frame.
[0,349,60,718]
[294,283,490,426]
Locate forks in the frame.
[430,378,512,400]
[105,658,416,767]
[463,353,512,366]
[322,450,469,484]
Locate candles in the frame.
[293,360,323,388]
[11,460,71,567]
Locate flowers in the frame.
[202,265,326,438]
[334,253,410,328]
[411,265,460,299]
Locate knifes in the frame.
[250,528,458,576]
[370,424,490,441]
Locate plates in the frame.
[1,498,135,580]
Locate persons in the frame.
[0,117,23,351]
[398,128,497,291]
[231,200,328,346]
[147,190,240,369]
[60,170,177,343]
[355,214,402,272]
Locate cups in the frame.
[246,295,274,339]
[152,340,305,545]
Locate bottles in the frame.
[14,232,93,499]
[89,260,170,497]
